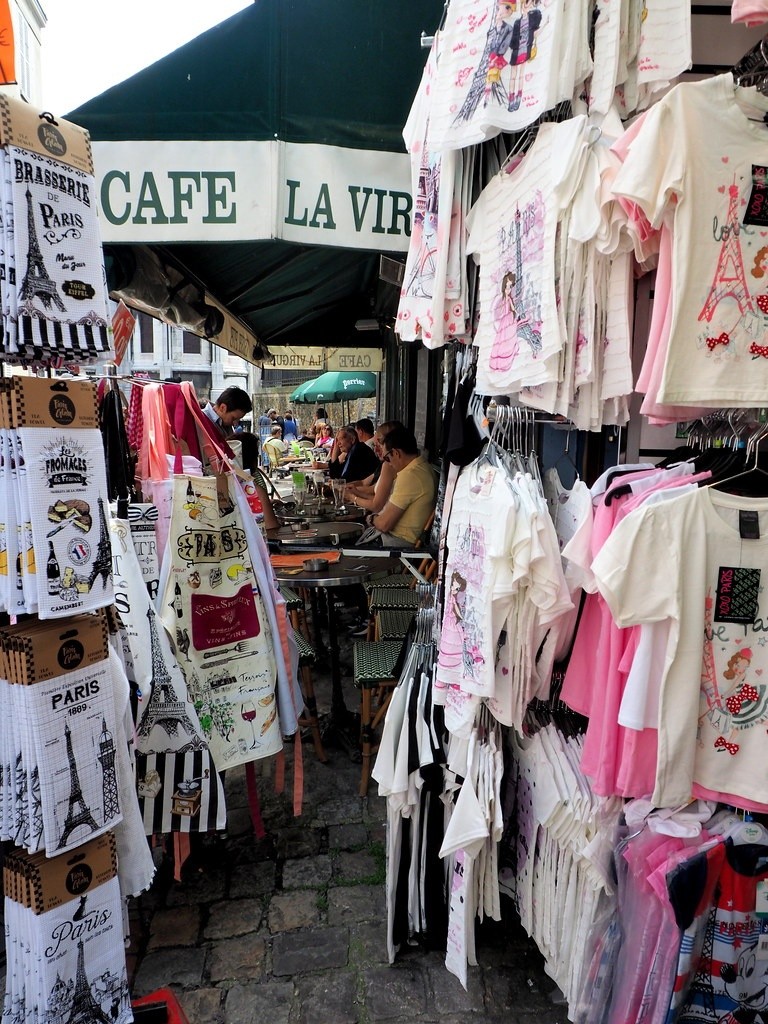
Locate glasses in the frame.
[323,427,331,431]
[382,450,394,463]
[372,443,381,448]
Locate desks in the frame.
[282,491,348,506]
[279,457,310,464]
[278,521,366,636]
[275,504,368,522]
[267,522,365,630]
[287,460,325,468]
[274,546,415,750]
[297,468,329,473]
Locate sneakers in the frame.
[347,609,372,635]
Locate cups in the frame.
[293,483,306,514]
[332,479,346,510]
[320,453,326,462]
[314,472,325,499]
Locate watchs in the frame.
[366,513,379,527]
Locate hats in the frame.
[267,409,276,418]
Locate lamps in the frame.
[355,319,380,331]
[378,254,405,287]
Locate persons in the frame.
[258,407,298,467]
[302,408,438,549]
[226,433,282,531]
[200,385,254,439]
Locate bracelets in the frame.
[353,496,358,506]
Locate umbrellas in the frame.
[290,371,377,426]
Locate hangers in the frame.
[605,408,768,506]
[473,404,545,498]
[703,800,768,847]
[732,36,768,124]
[396,585,438,687]
[619,798,696,841]
[500,100,602,178]
[477,672,584,745]
[553,419,583,482]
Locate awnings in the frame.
[59,0,448,373]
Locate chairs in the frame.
[264,443,291,478]
[293,627,329,765]
[299,440,314,448]
[368,560,437,641]
[361,558,429,607]
[352,640,402,799]
[279,585,303,631]
[370,577,438,706]
